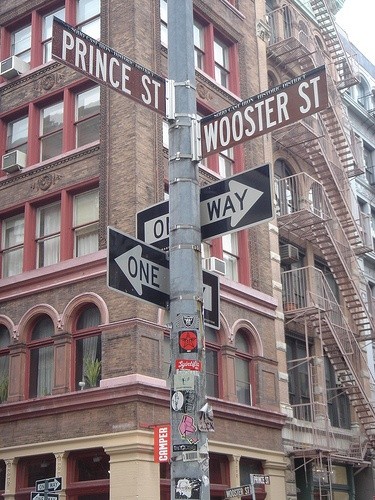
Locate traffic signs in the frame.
[200,64,329,159]
[107,226,220,331]
[34,477,62,492]
[251,475,270,485]
[32,492,59,500]
[225,485,251,498]
[135,162,274,251]
[52,16,165,119]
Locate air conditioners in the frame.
[337,368,355,386]
[207,254,228,274]
[0,148,28,172]
[281,242,300,262]
[0,54,30,79]
